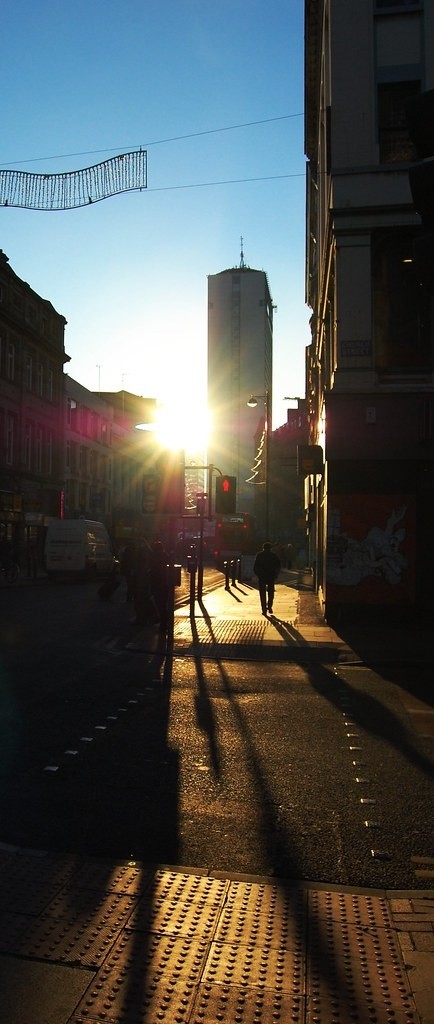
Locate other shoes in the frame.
[262,613,266,616]
[268,606,273,613]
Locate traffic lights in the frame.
[216,475,237,514]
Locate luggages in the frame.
[97,573,123,600]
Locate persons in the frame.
[123,539,168,628]
[0,534,27,577]
[254,542,281,615]
[287,544,295,570]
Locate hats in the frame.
[262,542,272,549]
